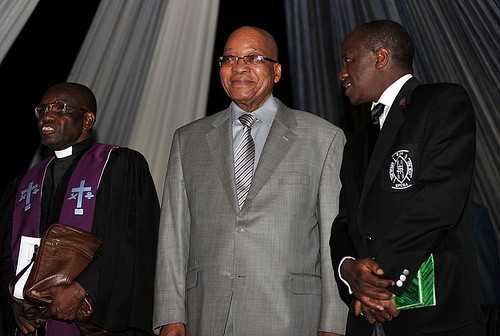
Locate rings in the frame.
[386,314,391,320]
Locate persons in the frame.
[152,24,353,336]
[0,82,161,336]
[328,21,500,336]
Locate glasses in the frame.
[219,56,278,67]
[35,101,89,119]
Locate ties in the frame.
[368,102,384,165]
[234,114,257,211]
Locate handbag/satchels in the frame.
[8,224,102,321]
